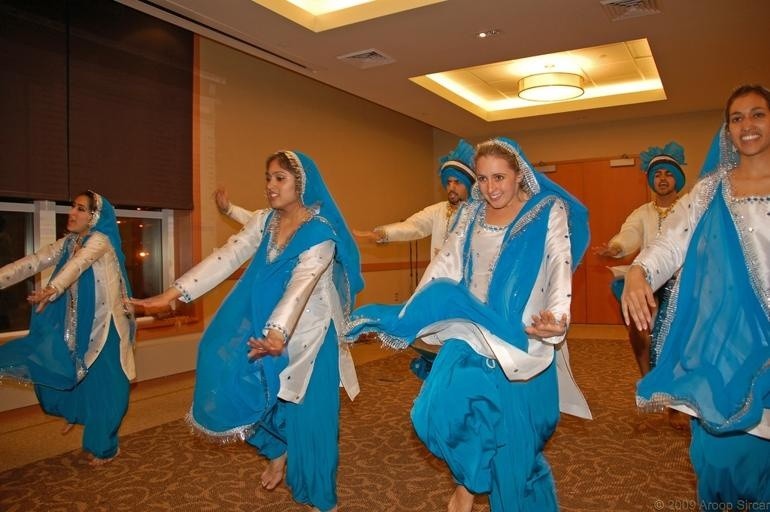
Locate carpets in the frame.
[1,336,702,512]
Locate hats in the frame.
[637,141,688,193]
[439,139,475,196]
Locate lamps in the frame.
[517,71,585,103]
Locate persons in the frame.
[620,82,770,511]
[121,150,368,512]
[0,187,137,468]
[335,137,594,512]
[588,140,689,432]
[350,139,486,264]
[211,186,263,222]
